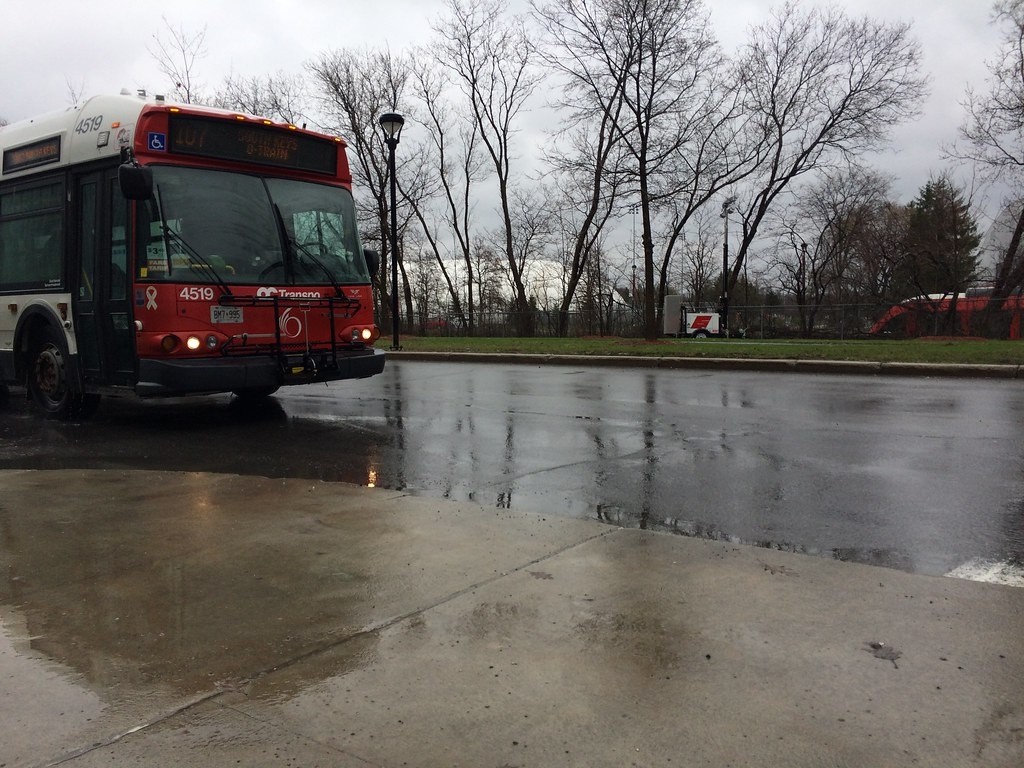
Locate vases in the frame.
[427,328,439,336]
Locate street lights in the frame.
[721,195,738,338]
[377,112,404,351]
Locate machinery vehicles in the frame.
[662,293,721,342]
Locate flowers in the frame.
[423,318,447,330]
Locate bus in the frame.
[0,91,385,416]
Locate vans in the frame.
[871,290,1024,342]
